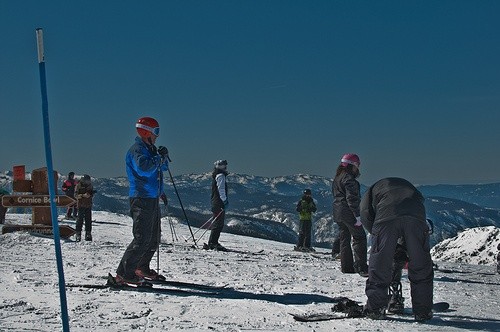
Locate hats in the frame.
[214,160,227,170]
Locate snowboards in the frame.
[288,302,449,321]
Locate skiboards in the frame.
[201,242,266,255]
[65,274,223,299]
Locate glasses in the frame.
[136,123,160,137]
[218,160,227,165]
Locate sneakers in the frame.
[85,234,92,241]
[415,312,433,322]
[72,216,78,220]
[116,275,153,288]
[296,246,315,252]
[208,242,228,251]
[342,263,359,273]
[76,235,81,242]
[363,305,386,319]
[135,267,165,282]
[65,215,73,220]
[357,265,369,277]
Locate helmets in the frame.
[341,153,360,168]
[304,189,311,194]
[136,117,160,140]
[81,175,90,181]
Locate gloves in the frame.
[224,200,228,209]
[156,146,169,164]
[354,216,361,226]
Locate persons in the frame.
[360,177,434,322]
[0,188,11,224]
[61,172,77,220]
[295,189,317,251]
[73,174,93,242]
[331,221,341,259]
[207,160,228,250]
[332,153,369,277]
[115,117,169,284]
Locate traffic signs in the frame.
[2,195,76,207]
[3,225,77,239]
